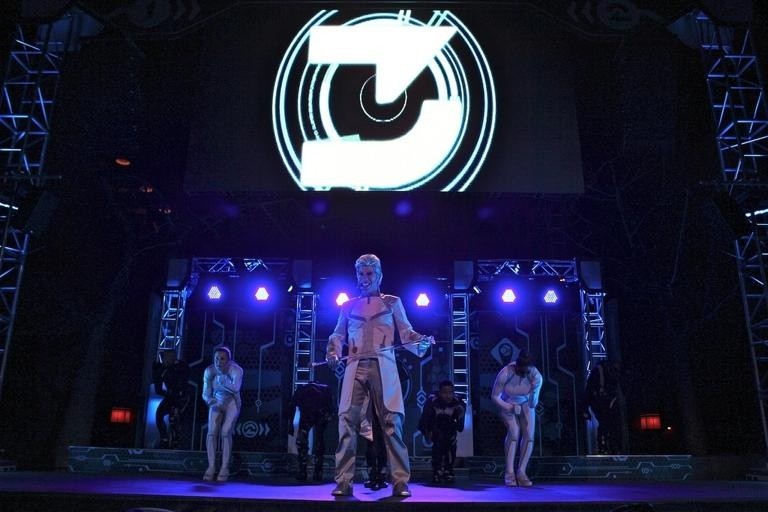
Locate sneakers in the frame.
[432,469,456,483]
[503,471,533,487]
[202,467,231,481]
[331,481,353,496]
[364,479,389,488]
[392,481,411,497]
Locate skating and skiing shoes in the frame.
[294,468,326,482]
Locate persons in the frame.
[415,379,467,486]
[363,412,389,490]
[323,252,435,498]
[153,348,199,449]
[488,354,544,488]
[580,357,629,455]
[198,346,244,484]
[287,382,333,482]
